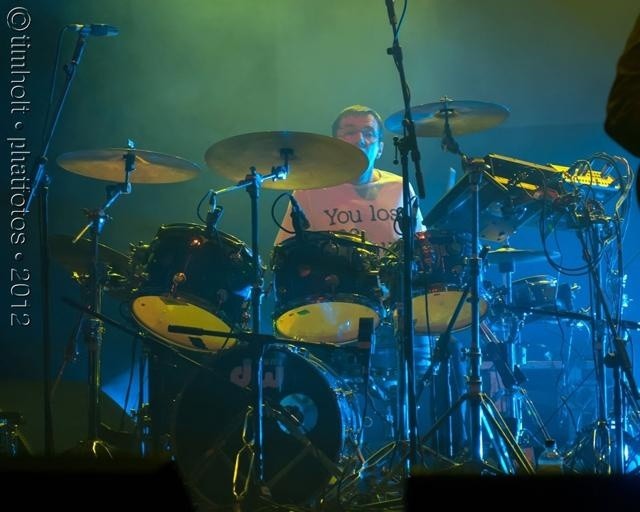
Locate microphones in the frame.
[69,23,120,39]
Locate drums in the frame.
[271,230,389,347]
[511,274,557,310]
[130,223,263,356]
[172,338,364,511]
[380,229,491,335]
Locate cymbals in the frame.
[42,230,134,303]
[385,99,512,139]
[205,130,369,191]
[56,147,204,185]
[483,246,558,262]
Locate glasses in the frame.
[334,129,380,144]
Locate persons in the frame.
[602,11,640,208]
[273,104,437,468]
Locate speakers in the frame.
[0,465,193,511]
